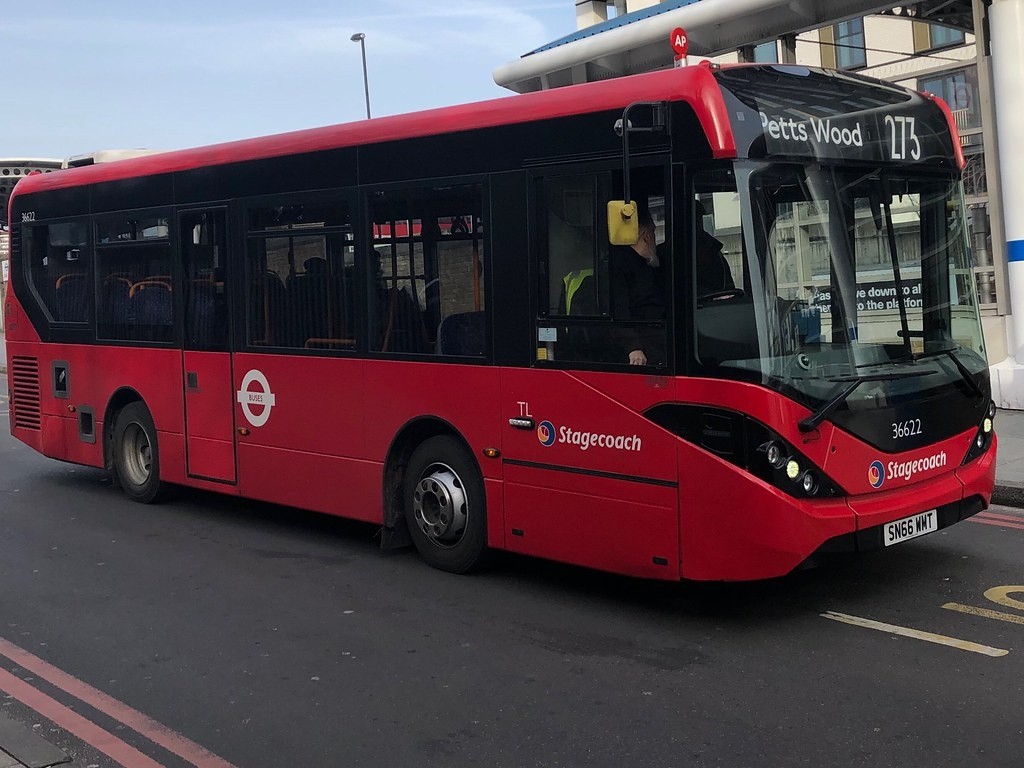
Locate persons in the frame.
[657,198,737,364]
[569,208,668,365]
[234,249,410,350]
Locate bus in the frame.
[3,64,997,583]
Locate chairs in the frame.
[104,277,134,322]
[55,273,88,321]
[285,272,306,292]
[185,278,219,341]
[557,268,594,349]
[127,280,172,324]
[434,309,485,355]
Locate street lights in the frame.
[350,33,370,119]
